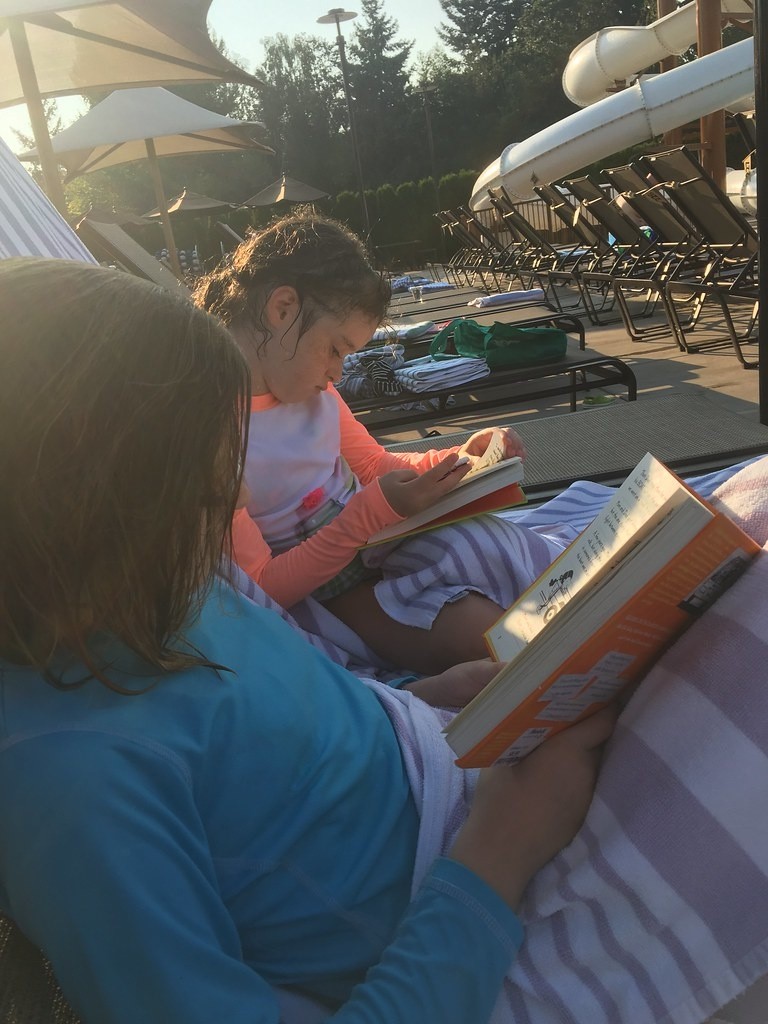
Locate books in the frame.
[440,451,765,768]
[356,430,528,549]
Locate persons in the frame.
[0,216,768,1024]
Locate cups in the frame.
[408,286,423,304]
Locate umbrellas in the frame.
[1,0,328,283]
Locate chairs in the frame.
[1,137,642,657]
[431,145,759,368]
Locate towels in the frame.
[393,358,492,393]
[355,454,768,1022]
[465,287,546,310]
[0,139,404,684]
[391,272,455,296]
[371,319,435,342]
[343,340,406,372]
